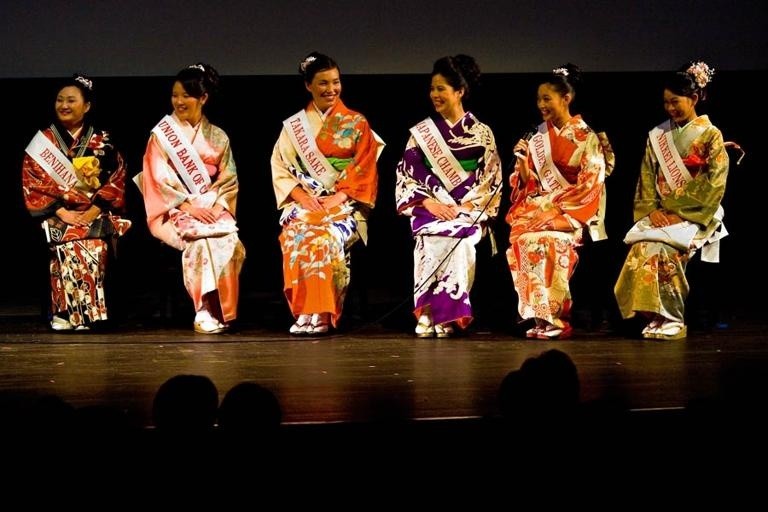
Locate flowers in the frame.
[511,125,538,163]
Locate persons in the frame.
[270,51,388,341]
[613,56,731,344]
[130,62,248,336]
[504,66,616,339]
[21,72,134,333]
[0,350,767,512]
[396,55,504,340]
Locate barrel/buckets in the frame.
[526,324,572,338]
[435,323,455,338]
[193,319,227,335]
[415,321,436,338]
[306,322,331,338]
[289,321,310,336]
[641,323,687,341]
